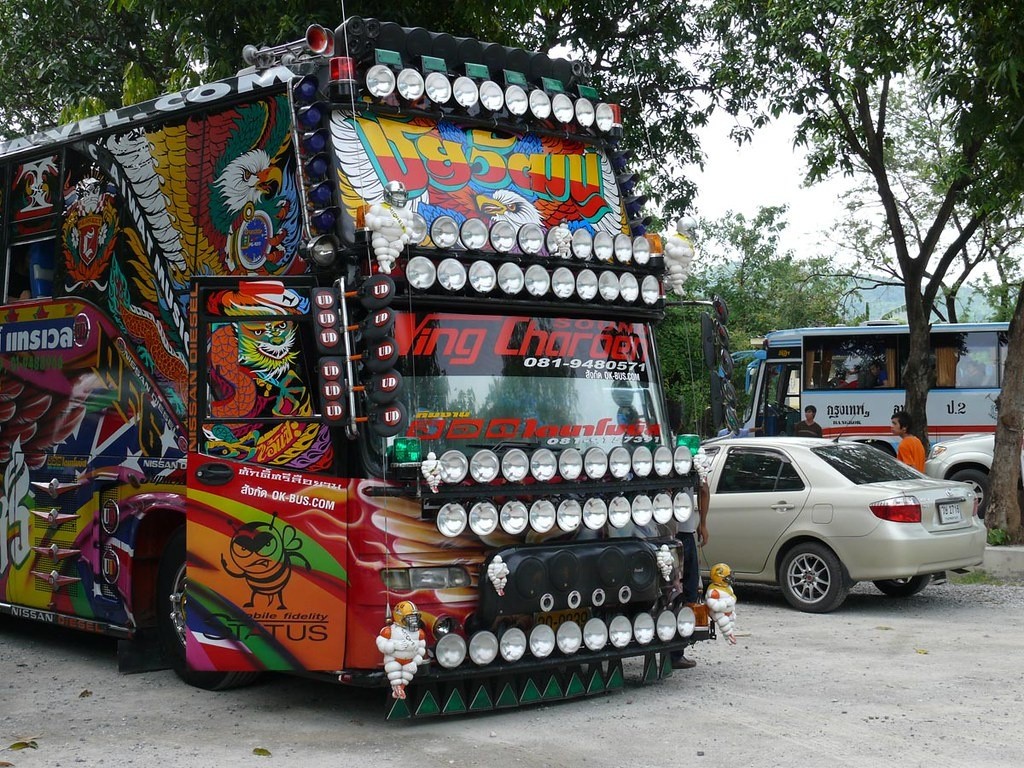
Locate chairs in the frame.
[27,242,56,299]
[955,362,997,388]
[724,464,776,492]
[859,371,878,390]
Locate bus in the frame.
[0,16,742,690]
[720,320,1010,460]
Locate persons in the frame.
[837,362,861,389]
[868,361,888,387]
[717,413,762,439]
[662,400,710,670]
[793,405,822,438]
[891,411,947,585]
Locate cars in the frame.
[692,436,986,611]
[924,430,1024,518]
